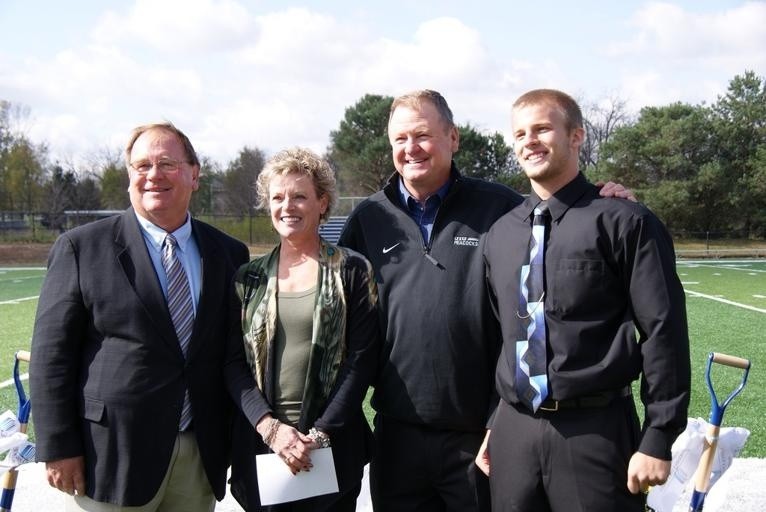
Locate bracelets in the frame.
[308,428,331,448]
[263,418,279,446]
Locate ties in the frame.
[515,203,548,415]
[160,233,194,433]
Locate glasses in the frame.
[129,159,188,174]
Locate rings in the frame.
[642,489,649,494]
[284,456,290,465]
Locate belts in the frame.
[537,389,633,414]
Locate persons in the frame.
[29,121,248,512]
[475,89,691,512]
[340,86,638,512]
[227,146,378,512]
